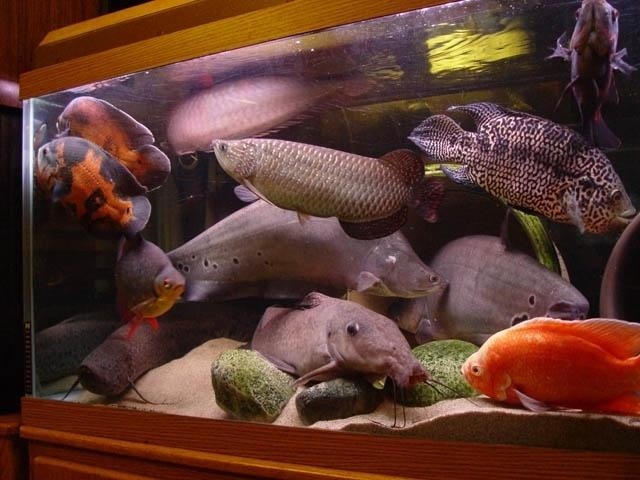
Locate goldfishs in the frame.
[461,317,640,416]
